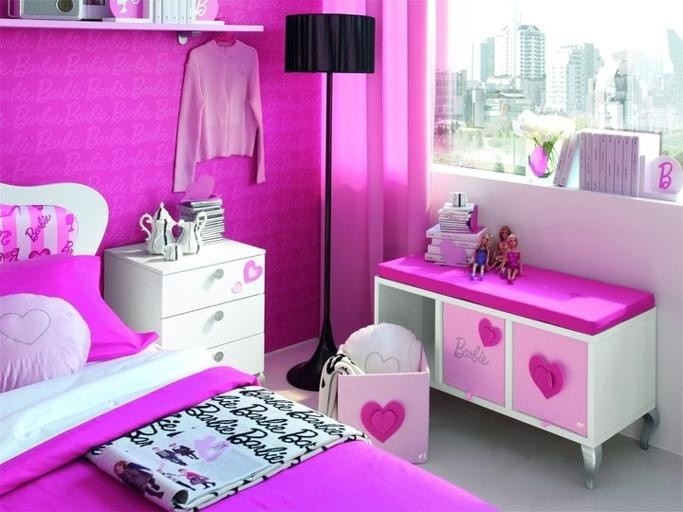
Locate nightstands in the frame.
[103,243,266,378]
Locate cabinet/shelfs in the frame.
[374,252,661,491]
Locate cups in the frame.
[161,243,183,262]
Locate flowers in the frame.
[512,109,577,178]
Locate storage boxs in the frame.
[337,342,430,465]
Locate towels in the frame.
[316,354,367,420]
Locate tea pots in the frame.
[140,202,177,256]
[176,211,206,255]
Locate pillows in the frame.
[0,294,90,393]
[344,324,422,374]
[0,253,158,364]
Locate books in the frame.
[175,195,226,244]
[551,128,659,198]
[153,0,193,24]
[423,200,496,269]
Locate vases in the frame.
[527,142,559,179]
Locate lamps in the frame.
[284,13,375,393]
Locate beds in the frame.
[0,181,504,512]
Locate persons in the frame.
[467,232,492,281]
[500,234,523,285]
[484,226,510,272]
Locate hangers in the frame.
[215,32,234,44]
